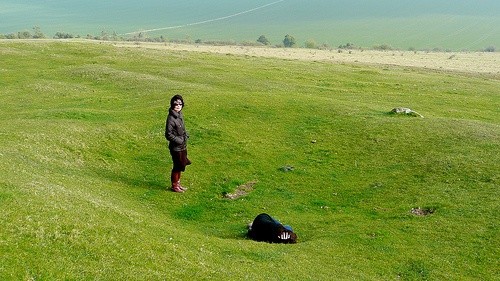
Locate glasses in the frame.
[174,104,183,106]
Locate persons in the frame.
[165,94,191,194]
[246,213,297,244]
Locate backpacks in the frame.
[249,214,297,244]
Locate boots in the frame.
[178,173,187,190]
[171,170,183,192]
